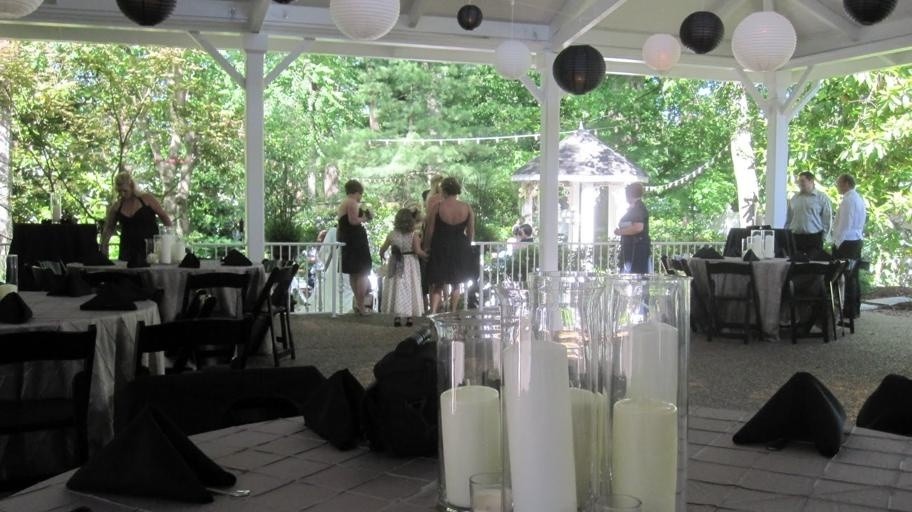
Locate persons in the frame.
[380,208,429,326]
[825,174,866,320]
[100,171,172,262]
[784,172,832,259]
[420,177,480,316]
[492,224,521,258]
[518,224,533,242]
[614,182,652,274]
[306,247,326,298]
[423,175,444,214]
[336,180,373,316]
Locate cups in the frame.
[425,270,695,510]
[145,226,194,265]
[51,193,62,225]
[0,254,19,301]
[741,229,775,258]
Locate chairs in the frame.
[133,319,241,368]
[261,258,295,272]
[255,264,300,365]
[660,255,715,333]
[704,260,763,344]
[180,269,252,317]
[1,324,98,476]
[793,259,850,341]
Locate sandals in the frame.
[405,318,413,327]
[394,317,401,327]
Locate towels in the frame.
[787,250,810,262]
[126,249,151,267]
[304,369,377,452]
[80,283,137,311]
[66,401,237,503]
[83,250,106,266]
[0,293,33,324]
[733,372,848,459]
[46,271,92,297]
[744,249,760,261]
[856,373,912,437]
[701,247,724,259]
[220,249,253,266]
[96,279,161,305]
[179,253,200,268]
[811,249,833,261]
[693,247,705,257]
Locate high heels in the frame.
[354,304,371,316]
